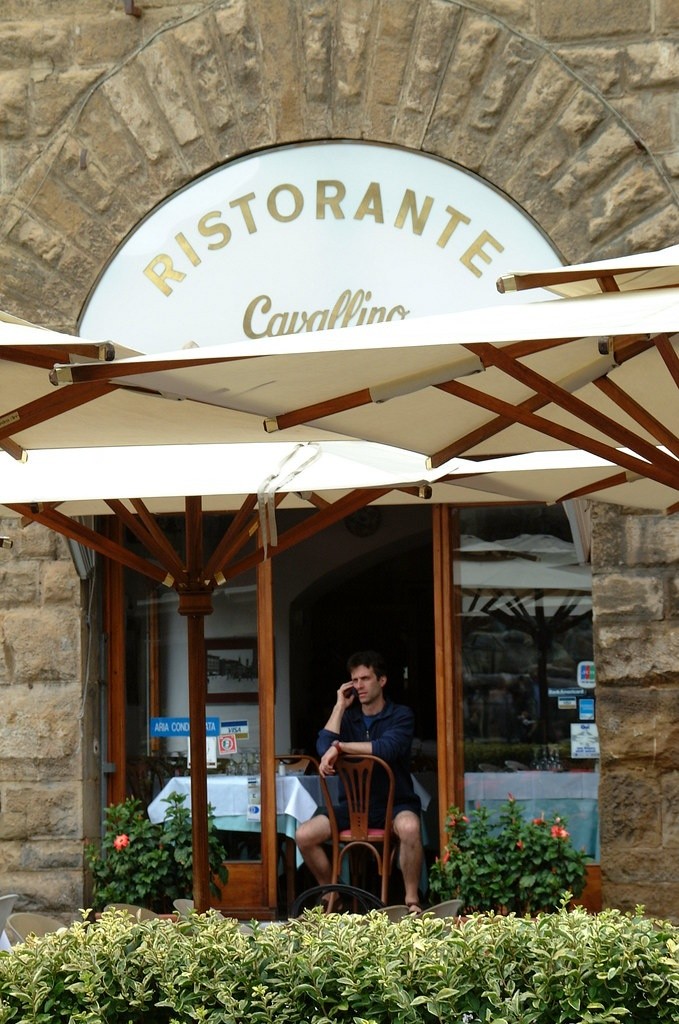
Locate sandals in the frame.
[406,902,421,914]
[319,897,343,912]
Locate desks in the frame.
[464,771,597,858]
[147,774,431,919]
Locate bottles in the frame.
[226,754,260,775]
[278,762,286,776]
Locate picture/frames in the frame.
[205,637,276,703]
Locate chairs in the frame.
[320,755,399,915]
[0,894,224,954]
[290,884,464,924]
[478,760,529,772]
[272,755,319,776]
[125,755,164,819]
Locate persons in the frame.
[295,650,423,920]
[462,663,541,742]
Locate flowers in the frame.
[427,795,596,920]
[81,791,229,915]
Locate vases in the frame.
[95,912,179,923]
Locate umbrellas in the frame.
[0,242,679,517]
[0,440,677,904]
[452,534,593,698]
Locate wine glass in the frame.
[529,744,564,770]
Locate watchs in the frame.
[330,740,342,754]
